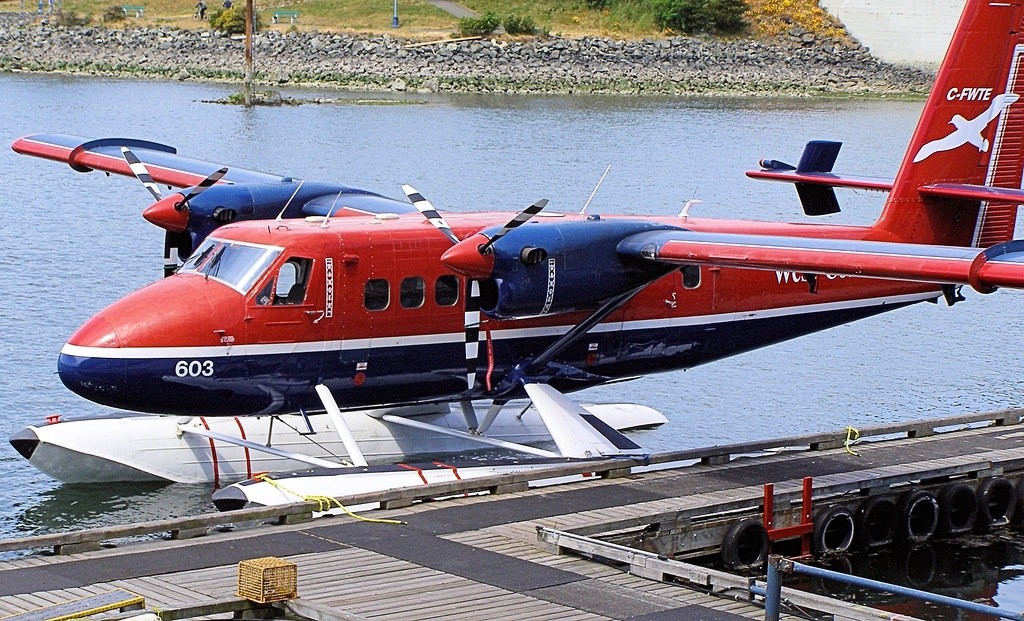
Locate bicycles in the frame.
[194,6,209,21]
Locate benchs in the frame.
[0,587,146,621]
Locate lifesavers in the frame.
[895,544,938,589]
[720,518,771,570]
[940,544,985,588]
[815,556,853,596]
[853,494,901,546]
[895,488,941,544]
[977,478,1018,524]
[941,485,979,531]
[1016,478,1024,518]
[810,505,858,555]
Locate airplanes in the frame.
[8,0,1024,529]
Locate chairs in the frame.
[286,283,305,303]
